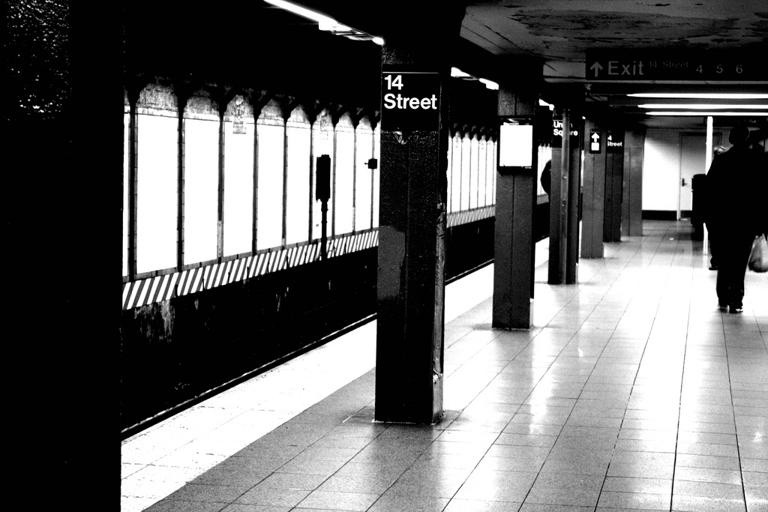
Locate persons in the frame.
[540,158,551,203]
[715,123,768,314]
[699,146,730,270]
